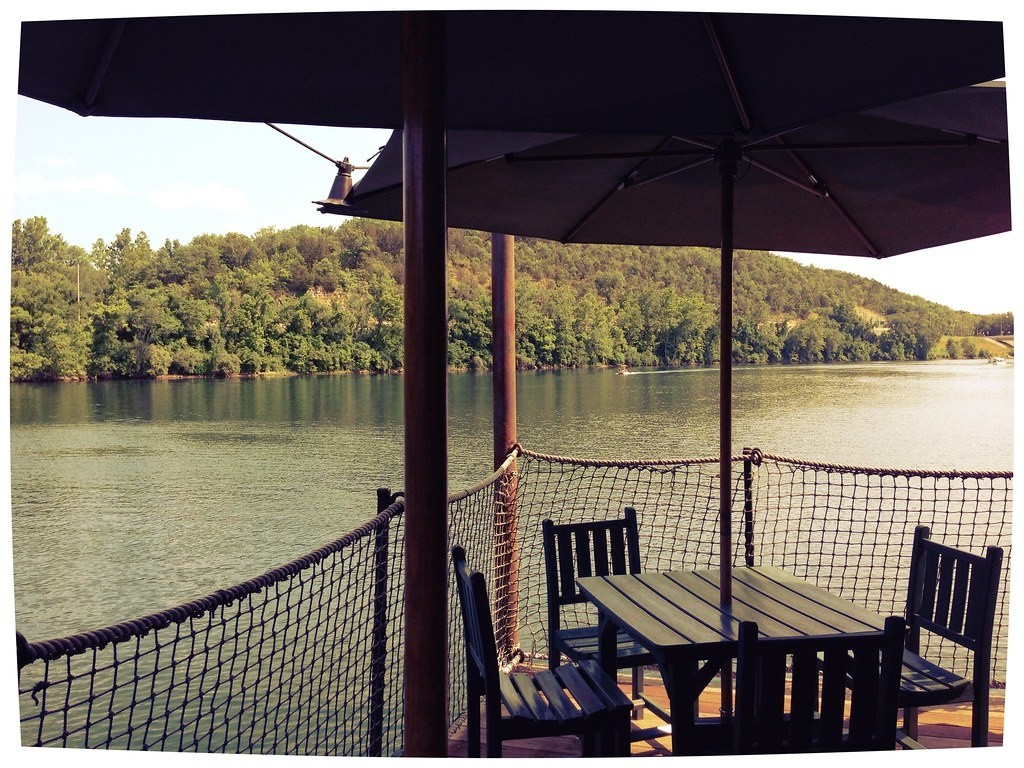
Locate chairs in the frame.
[693,614,906,750]
[451,543,635,757]
[846,525,1004,747]
[541,507,673,744]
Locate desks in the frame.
[576,564,911,743]
